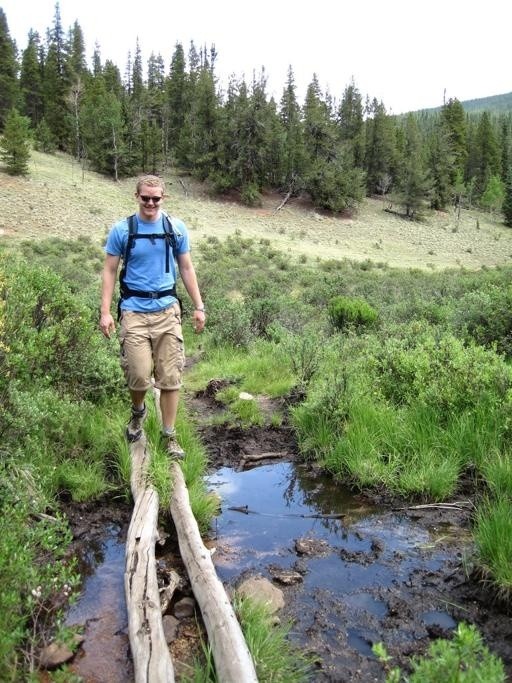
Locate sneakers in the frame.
[160,432,185,459]
[126,403,147,442]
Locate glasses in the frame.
[141,196,161,202]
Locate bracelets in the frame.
[195,305,205,312]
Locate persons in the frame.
[97,174,206,458]
